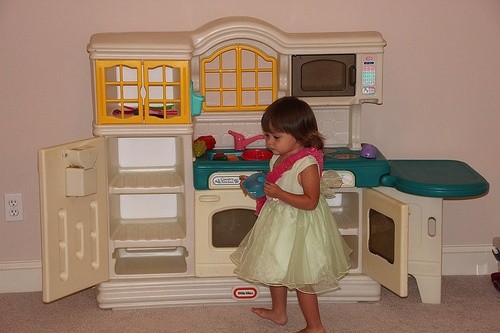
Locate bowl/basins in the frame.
[242,173,267,198]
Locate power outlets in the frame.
[5,193,23,221]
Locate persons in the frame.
[230,97,354,333]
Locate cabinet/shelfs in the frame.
[373,186,445,304]
[86,31,194,130]
[38,130,196,309]
[195,186,408,304]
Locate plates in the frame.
[242,149,273,161]
